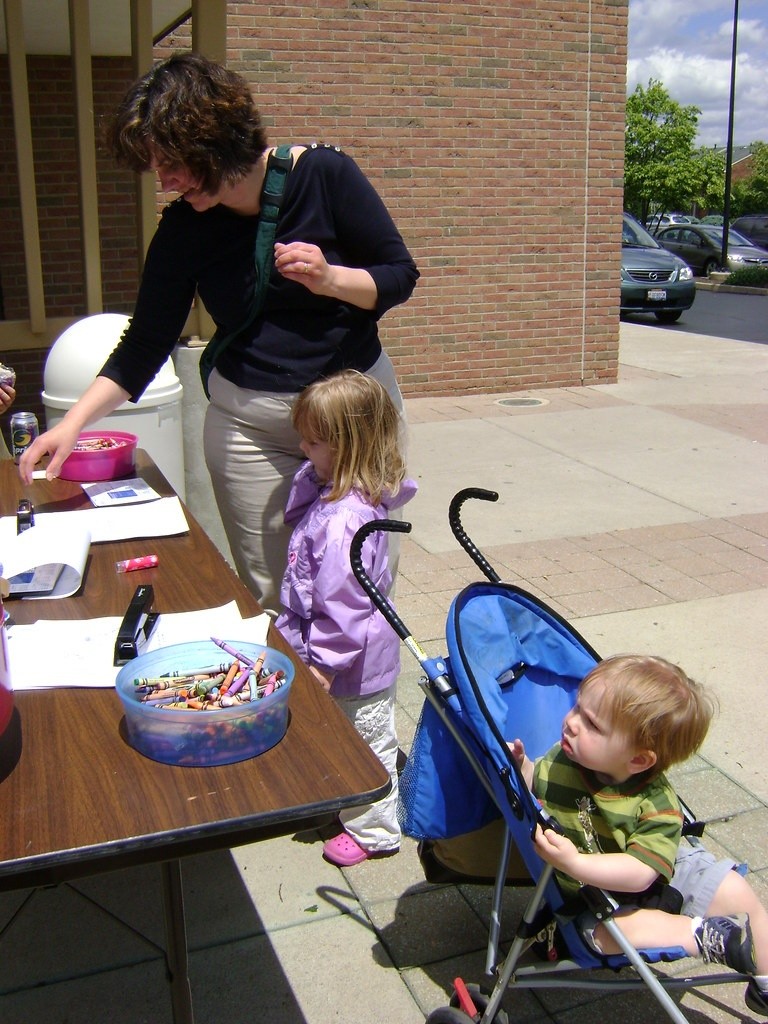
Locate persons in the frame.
[19,48,421,779]
[0,383,16,415]
[275,369,418,866]
[506,655,768,1017]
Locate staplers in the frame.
[17,500,34,535]
[113,584,163,665]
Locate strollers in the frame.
[348,485,768,1023]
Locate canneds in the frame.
[10,411,39,464]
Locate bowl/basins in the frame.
[116,639,296,767]
[49,431,138,481]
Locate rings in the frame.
[304,262,308,274]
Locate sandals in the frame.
[322,831,377,867]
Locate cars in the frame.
[636,213,691,238]
[619,212,696,322]
[650,224,768,279]
[728,215,768,251]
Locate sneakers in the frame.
[743,975,768,1018]
[695,910,761,979]
